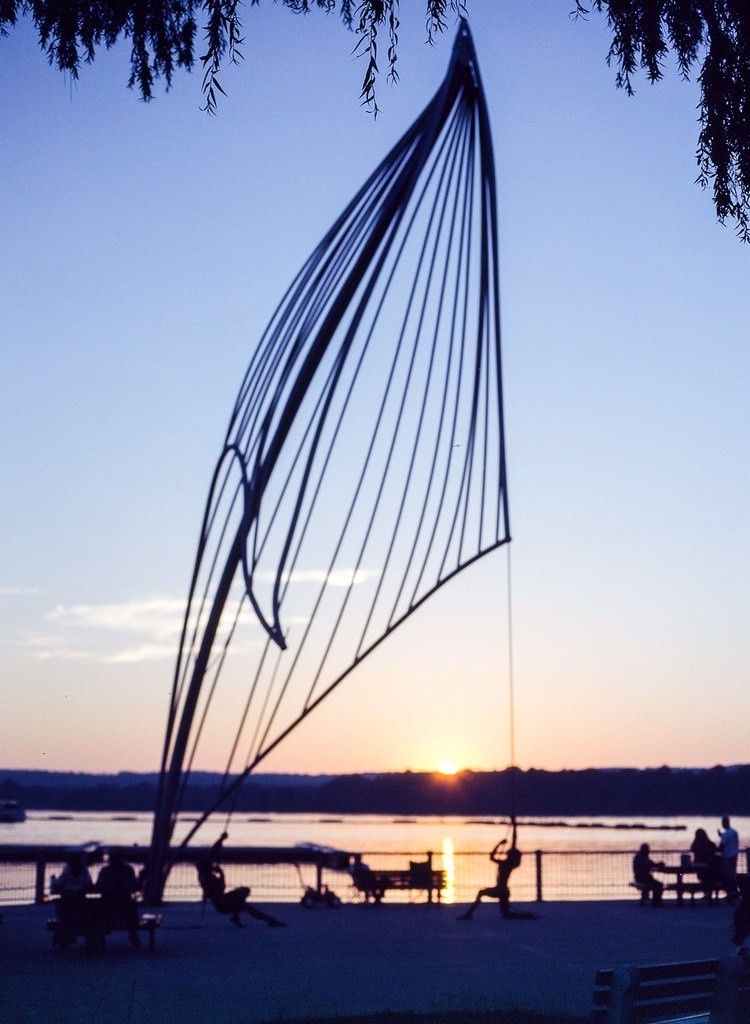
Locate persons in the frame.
[49,848,147,949]
[633,844,665,901]
[197,832,287,928]
[348,855,380,903]
[690,816,739,899]
[457,821,537,921]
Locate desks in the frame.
[657,867,698,900]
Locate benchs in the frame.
[47,912,163,951]
[589,946,750,1023]
[365,871,447,903]
[626,883,723,903]
